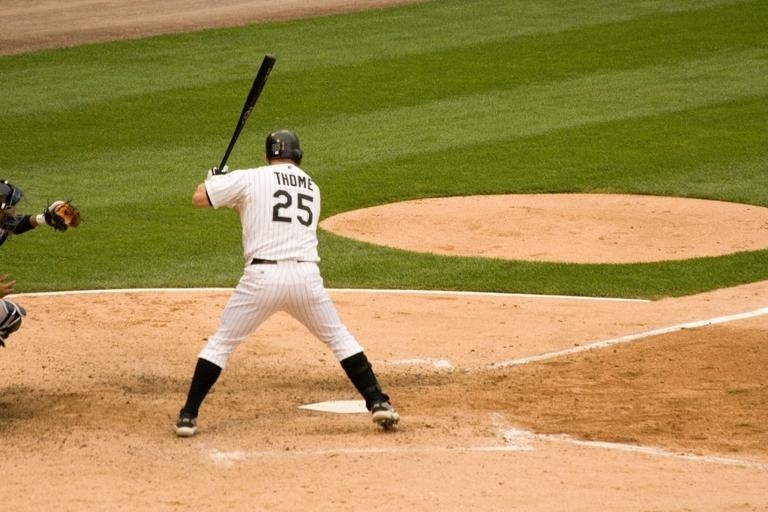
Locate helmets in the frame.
[265,129,303,166]
[0,180,28,241]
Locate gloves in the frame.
[207,165,228,179]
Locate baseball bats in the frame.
[217,55,276,175]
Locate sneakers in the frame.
[173,408,198,436]
[370,400,400,427]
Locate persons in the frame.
[175,129,400,438]
[1,178,81,346]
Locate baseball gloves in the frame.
[44,199,80,232]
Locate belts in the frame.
[251,258,304,264]
